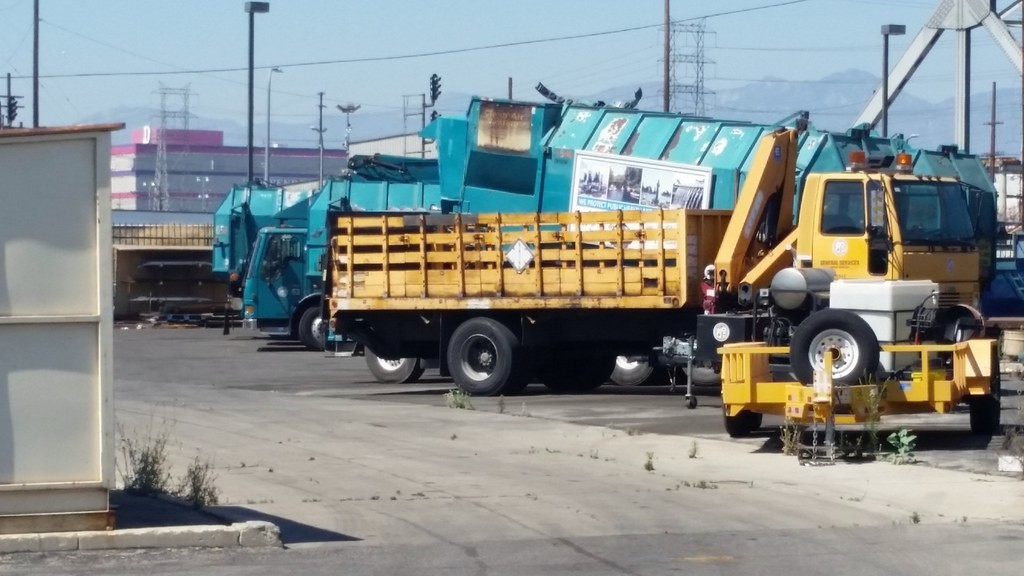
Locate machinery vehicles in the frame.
[422,80,998,291]
[206,153,435,349]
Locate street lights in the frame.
[337,103,361,151]
[881,22,907,136]
[310,126,328,191]
[243,0,269,181]
[265,67,285,182]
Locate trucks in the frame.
[323,133,983,397]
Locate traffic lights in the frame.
[431,73,442,99]
